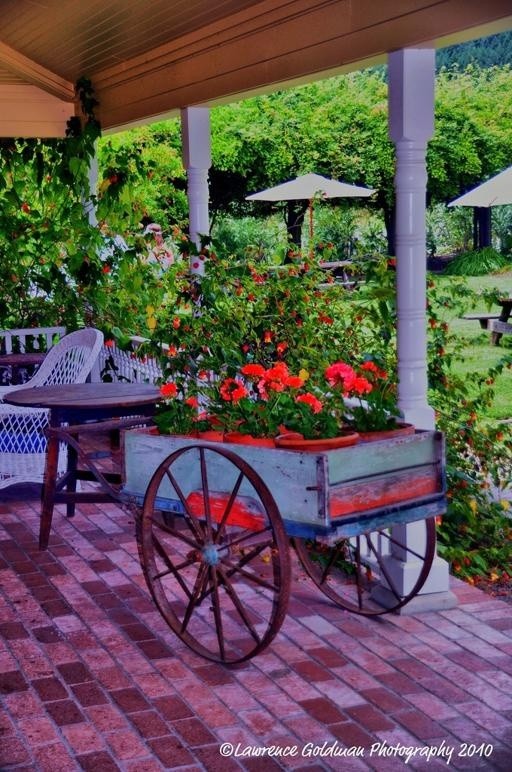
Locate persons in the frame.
[143,223,174,278]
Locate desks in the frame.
[3,382,185,551]
[490,297,512,346]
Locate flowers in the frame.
[150,262,395,422]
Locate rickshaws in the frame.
[45,416,448,664]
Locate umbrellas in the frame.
[245,172,376,282]
[448,165,512,208]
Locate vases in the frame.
[148,424,417,451]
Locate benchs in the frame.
[268,259,353,290]
[463,312,511,329]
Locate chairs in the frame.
[2,326,108,503]
